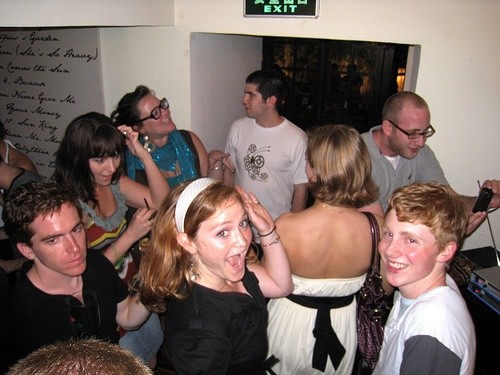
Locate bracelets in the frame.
[111,242,124,261]
[212,166,223,171]
[257,225,277,237]
[260,234,281,247]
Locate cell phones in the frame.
[472,188,494,213]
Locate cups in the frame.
[139,212,157,252]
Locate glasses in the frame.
[141,97,170,120]
[389,119,435,139]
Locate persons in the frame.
[0,157,42,228]
[137,178,294,375]
[360,91,500,237]
[0,181,151,375]
[370,183,476,375]
[0,120,38,174]
[5,337,152,375]
[248,125,394,375]
[52,112,171,369]
[110,86,234,266]
[223,69,310,260]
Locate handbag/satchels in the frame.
[356,211,394,370]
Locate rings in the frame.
[256,202,261,204]
